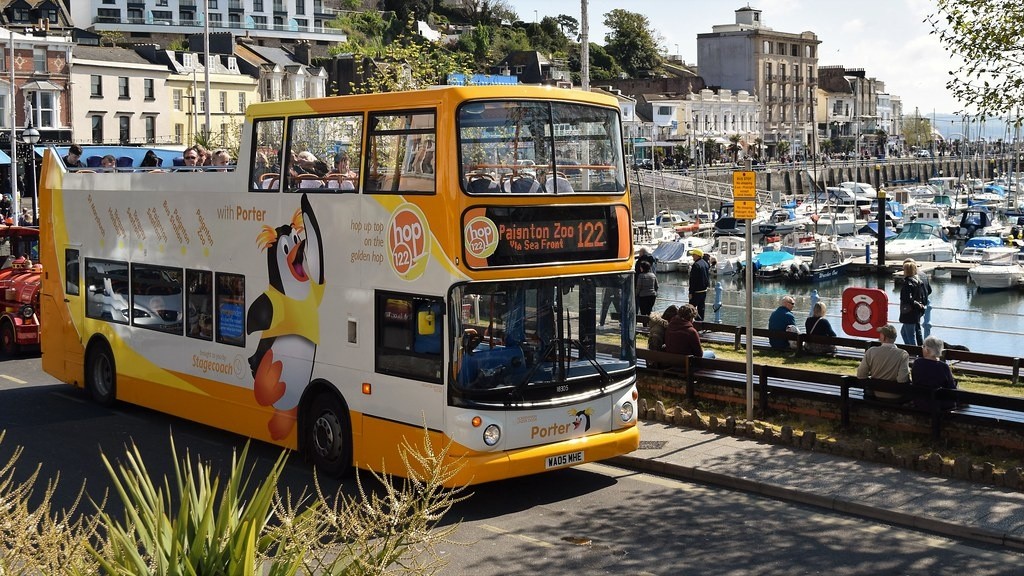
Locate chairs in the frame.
[257,173,360,191]
[87,153,187,170]
[465,168,571,194]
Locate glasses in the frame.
[922,345,926,349]
[787,301,795,305]
[184,156,197,159]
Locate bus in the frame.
[38,85,641,488]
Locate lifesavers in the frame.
[806,205,811,212]
[998,215,1007,224]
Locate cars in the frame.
[67,258,199,337]
[914,149,930,157]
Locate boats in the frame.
[632,170,1024,290]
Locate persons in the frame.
[62,142,234,173]
[688,248,709,321]
[596,248,659,331]
[0,173,33,227]
[268,147,358,188]
[899,258,932,347]
[781,155,800,164]
[768,295,837,357]
[856,326,957,410]
[647,303,715,371]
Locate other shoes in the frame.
[596,325,604,330]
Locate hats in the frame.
[689,249,703,258]
[876,325,896,340]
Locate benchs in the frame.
[560,308,1024,441]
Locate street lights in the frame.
[21,119,41,225]
